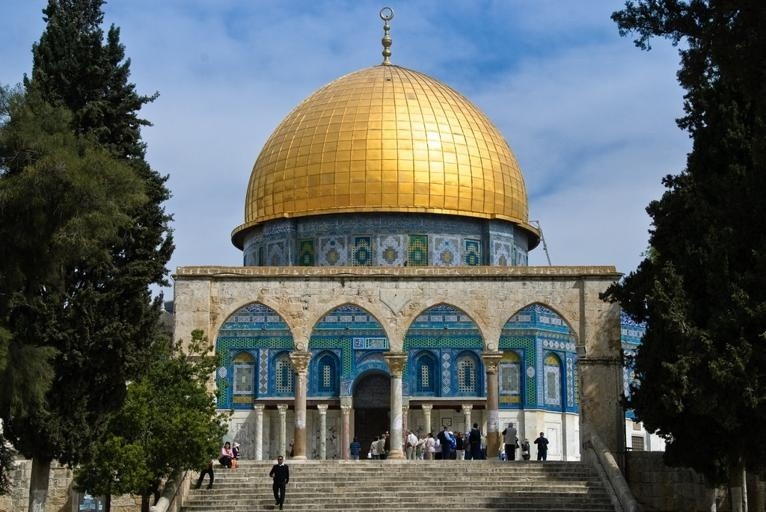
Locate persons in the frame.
[193,459,215,491]
[232,441,241,460]
[217,440,234,468]
[501,422,518,461]
[349,435,362,460]
[368,422,488,462]
[534,431,549,461]
[268,455,290,510]
[521,438,531,460]
